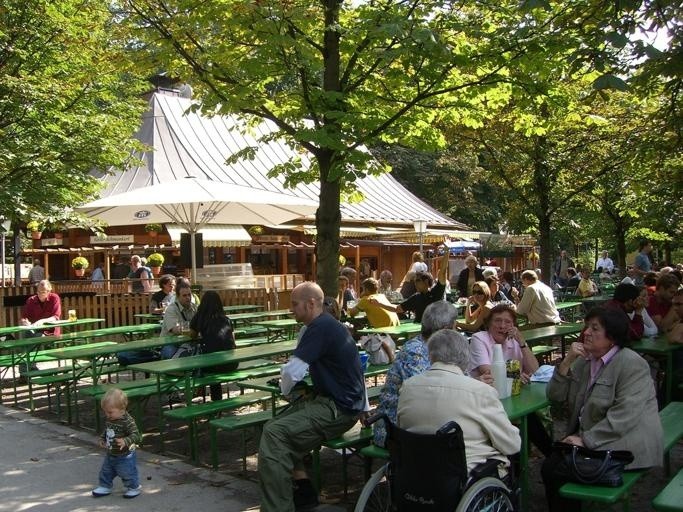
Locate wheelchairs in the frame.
[354,412,519,512]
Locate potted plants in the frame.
[145,253,164,274]
[338,255,346,272]
[27,221,45,239]
[530,253,539,266]
[144,223,162,236]
[51,223,66,238]
[71,257,90,276]
[249,226,263,241]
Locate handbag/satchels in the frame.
[551,441,634,487]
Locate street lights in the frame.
[412,214,427,255]
[497,220,508,271]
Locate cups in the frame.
[68,309,76,321]
[179,320,190,334]
[490,343,508,400]
[506,377,514,398]
[346,300,353,317]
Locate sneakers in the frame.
[92,487,111,495]
[123,486,142,498]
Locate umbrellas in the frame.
[74,176,320,238]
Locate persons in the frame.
[470,304,554,458]
[297,297,340,347]
[258,280,366,512]
[91,263,104,288]
[396,329,521,505]
[127,253,239,401]
[596,238,683,381]
[549,249,599,308]
[540,304,662,511]
[374,301,494,453]
[28,259,45,284]
[337,238,561,365]
[92,388,142,498]
[21,280,61,336]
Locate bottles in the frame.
[506,359,521,396]
[359,351,371,373]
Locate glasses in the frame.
[472,290,484,295]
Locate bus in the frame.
[0,235,34,279]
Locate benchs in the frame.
[1,273,683,512]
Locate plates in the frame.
[151,311,165,315]
